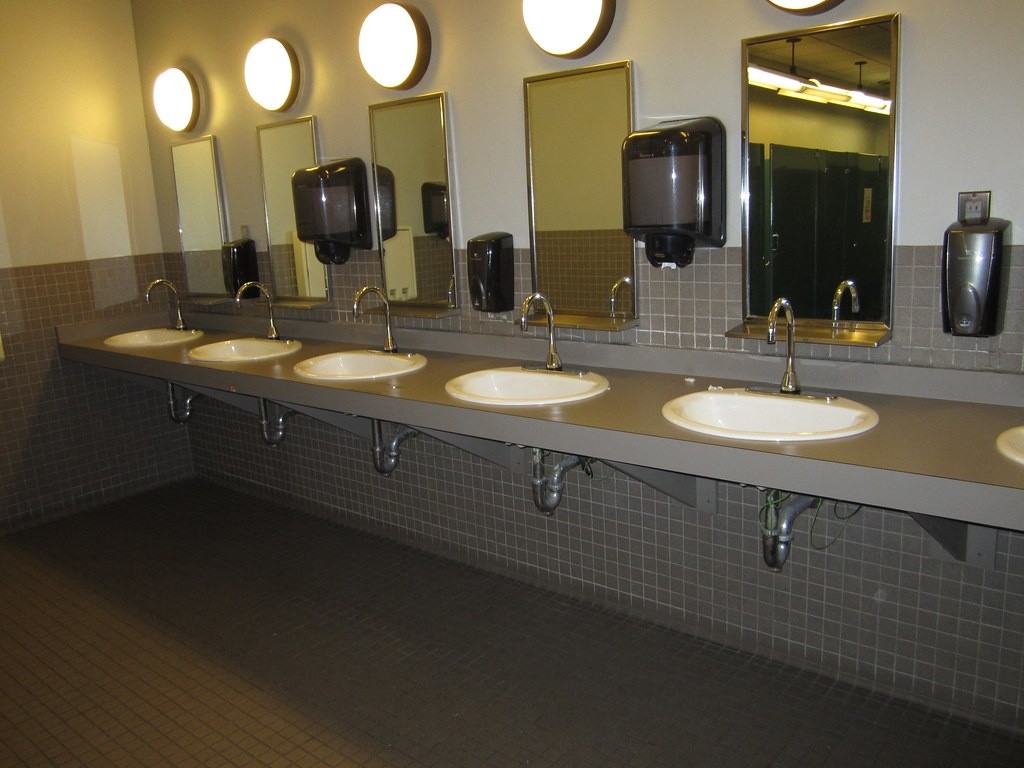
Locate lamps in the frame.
[244,35,301,114]
[767,0,844,17]
[747,38,891,116]
[357,2,432,91]
[521,0,616,60]
[151,66,201,135]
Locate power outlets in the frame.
[958,191,990,222]
[240,225,250,239]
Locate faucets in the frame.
[767,297,802,393]
[353,286,399,352]
[234,281,280,339]
[609,276,634,316]
[448,278,456,308]
[831,280,860,321]
[520,292,562,371]
[145,279,187,329]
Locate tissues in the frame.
[620,116,727,269]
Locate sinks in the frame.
[445,366,609,405]
[104,328,204,347]
[661,387,880,441]
[293,350,427,380]
[187,337,302,361]
[994,426,1024,467]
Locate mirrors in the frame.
[256,114,332,303]
[169,134,235,299]
[367,90,461,309]
[722,13,900,332]
[523,59,642,320]
